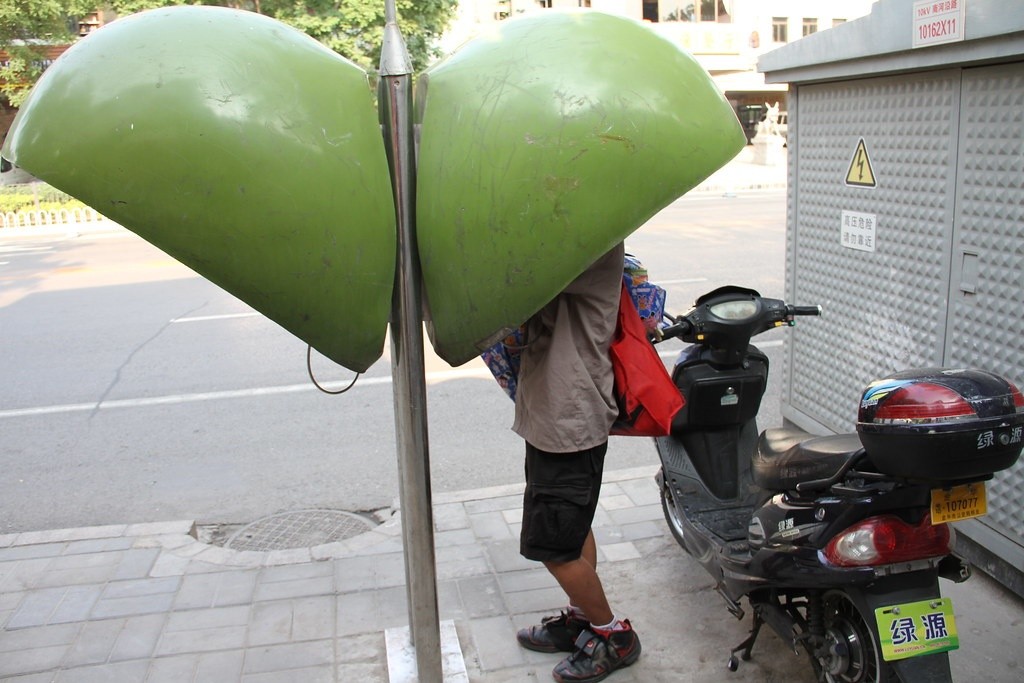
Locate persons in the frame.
[510,238,643,683]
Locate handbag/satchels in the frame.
[480,248,667,401]
[609,279,684,438]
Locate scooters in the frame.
[646,286,1024,683]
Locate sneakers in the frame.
[553,619,642,683]
[516,607,593,653]
